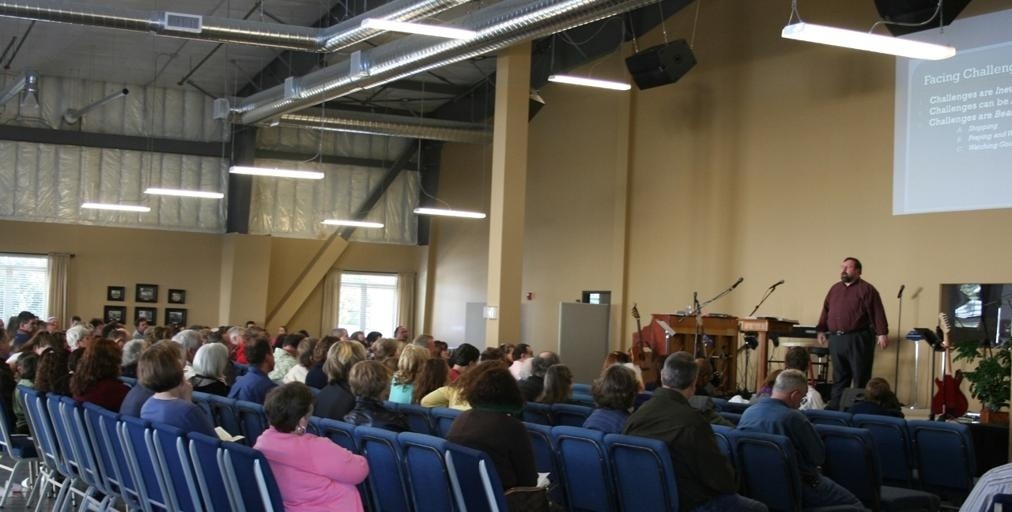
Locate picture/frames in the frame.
[104,284,187,326]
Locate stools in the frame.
[810,348,831,379]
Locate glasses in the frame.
[792,389,807,404]
[702,373,713,380]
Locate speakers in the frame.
[874,0,972,37]
[528,87,546,122]
[624,39,697,91]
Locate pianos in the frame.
[632,313,739,393]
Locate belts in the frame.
[831,330,857,336]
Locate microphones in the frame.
[770,280,784,289]
[692,291,697,308]
[733,276,743,288]
[897,284,906,298]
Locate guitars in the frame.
[631,303,657,391]
[932,311,968,419]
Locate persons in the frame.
[581,346,904,510]
[816,258,889,412]
[959,462,1012,512]
[0,311,578,510]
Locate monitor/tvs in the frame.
[939,283,1012,349]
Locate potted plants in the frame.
[952,338,1010,424]
